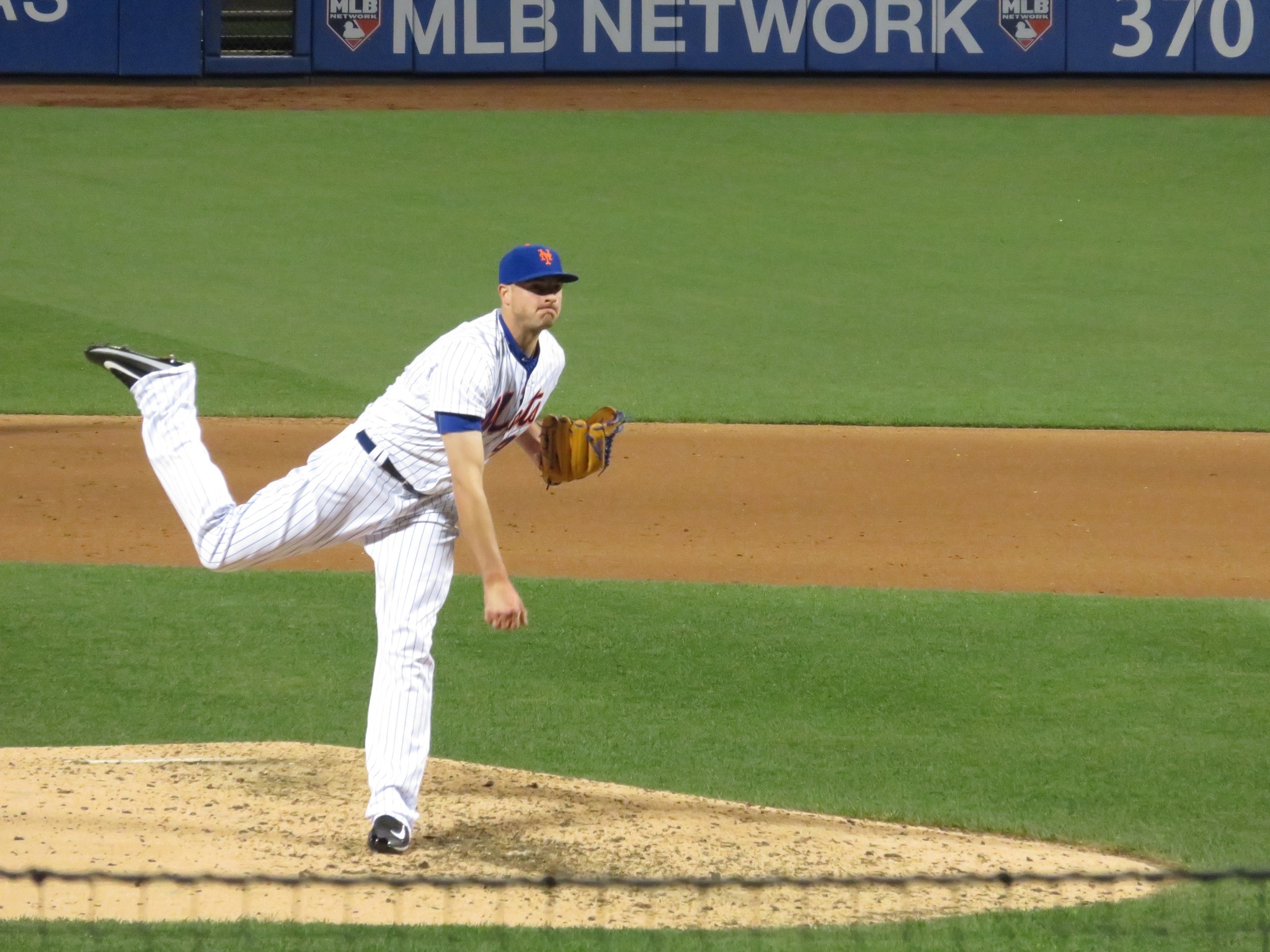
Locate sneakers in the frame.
[83,341,188,389]
[367,816,413,855]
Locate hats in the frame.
[498,242,579,285]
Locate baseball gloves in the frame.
[536,405,625,486]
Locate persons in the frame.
[85,244,579,853]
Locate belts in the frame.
[358,430,416,494]
